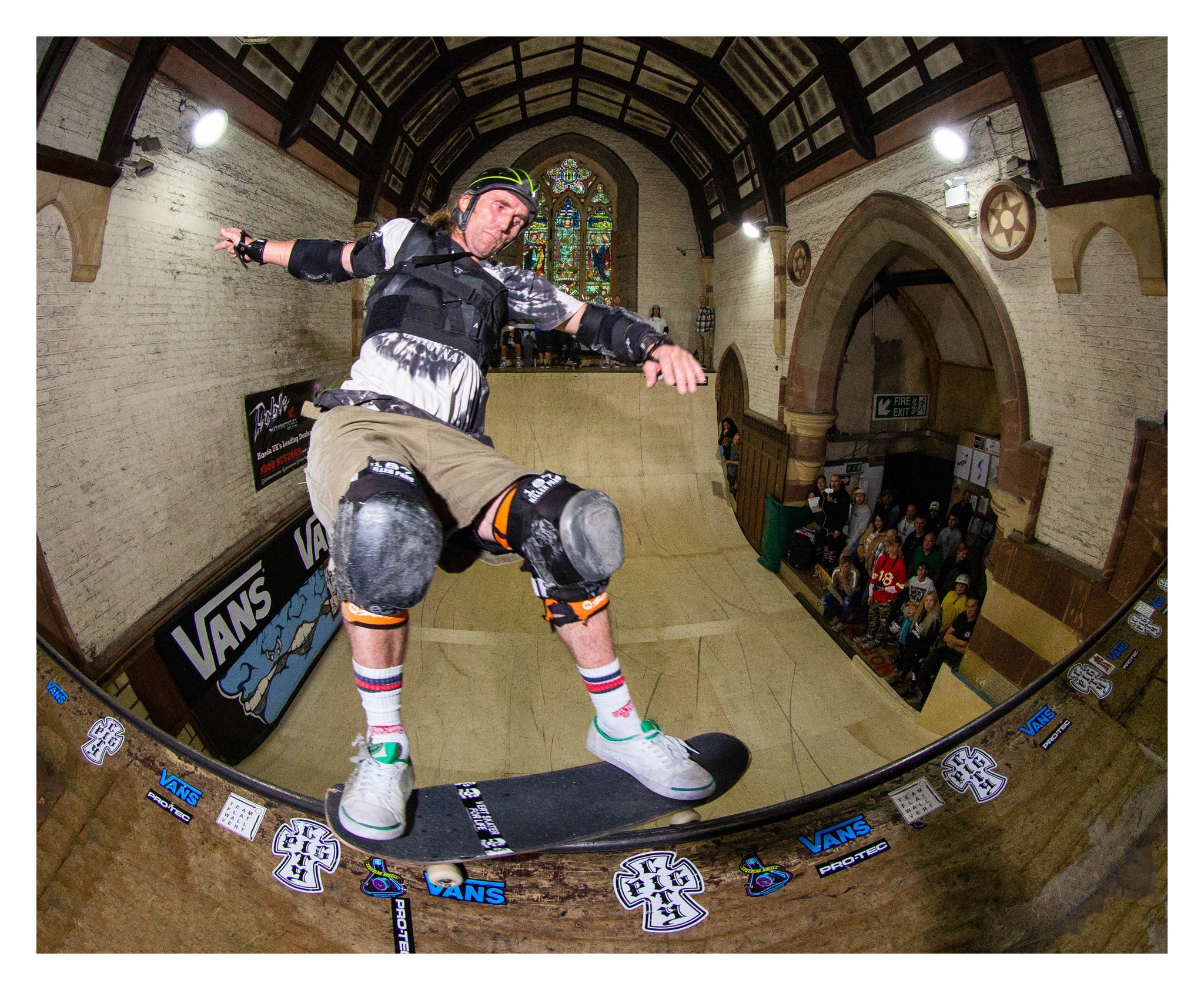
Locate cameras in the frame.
[873,580,883,592]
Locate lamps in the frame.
[134,135,164,152]
[1009,175,1041,193]
[176,100,229,149]
[930,126,970,164]
[1005,156,1030,175]
[122,158,154,178]
[741,221,763,240]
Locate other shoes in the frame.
[863,639,881,650]
[891,667,900,674]
[830,616,840,626]
[888,659,898,664]
[832,622,844,633]
[853,634,874,642]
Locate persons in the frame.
[695,295,715,369]
[717,417,739,492]
[791,471,992,708]
[535,328,555,369]
[601,294,624,368]
[648,305,669,336]
[722,433,741,497]
[211,163,719,839]
[565,339,580,368]
[498,323,524,368]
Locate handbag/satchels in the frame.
[886,636,898,646]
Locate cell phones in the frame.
[826,489,833,493]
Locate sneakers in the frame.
[337,730,416,840]
[900,688,915,699]
[586,715,717,799]
[889,678,905,686]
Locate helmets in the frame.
[466,166,542,224]
[955,574,971,589]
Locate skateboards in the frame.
[322,730,755,862]
[812,563,833,591]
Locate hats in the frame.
[853,489,865,495]
[929,501,940,511]
